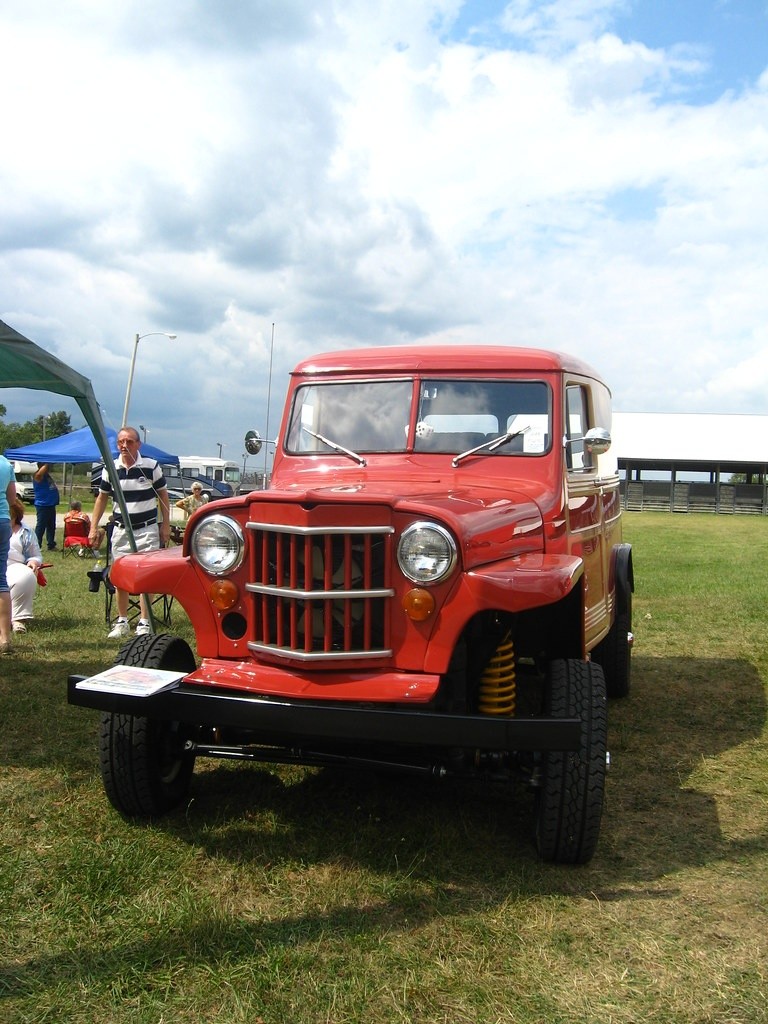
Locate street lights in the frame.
[139,425,149,444]
[43,415,51,442]
[241,452,249,484]
[217,443,223,458]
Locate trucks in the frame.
[7,459,40,505]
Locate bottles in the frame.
[88,560,102,592]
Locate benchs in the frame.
[425,432,524,451]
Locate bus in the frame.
[162,454,241,498]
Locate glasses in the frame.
[194,488,201,491]
[118,440,138,445]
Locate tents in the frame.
[3,423,181,464]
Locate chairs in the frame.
[62,516,97,558]
[167,525,185,548]
[31,563,52,601]
[87,522,175,631]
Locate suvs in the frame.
[66,343,634,865]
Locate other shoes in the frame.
[78,549,84,557]
[12,619,27,634]
[0,641,12,655]
[92,550,103,559]
[51,547,59,551]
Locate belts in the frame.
[115,519,156,530]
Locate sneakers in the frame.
[108,620,130,639]
[134,621,150,635]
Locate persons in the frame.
[174,481,208,514]
[0,453,44,654]
[30,460,60,552]
[63,499,106,561]
[86,425,172,639]
